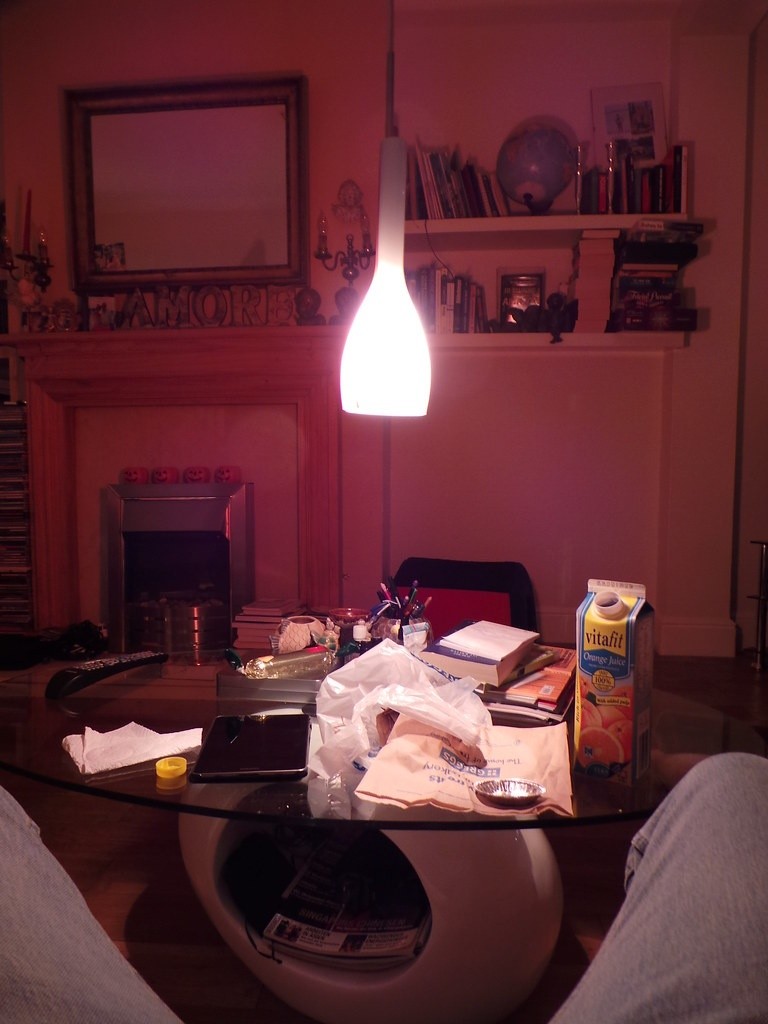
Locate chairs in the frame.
[390,557,541,642]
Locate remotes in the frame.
[45,650,168,700]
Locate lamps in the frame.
[317,205,377,325]
[337,0,434,420]
[0,228,52,331]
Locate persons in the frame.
[1,752,768,1024]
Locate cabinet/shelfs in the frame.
[392,210,692,358]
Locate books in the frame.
[262,839,431,970]
[416,141,508,220]
[496,267,546,323]
[568,221,704,333]
[418,620,574,721]
[0,405,32,623]
[232,598,332,649]
[576,141,688,215]
[406,266,485,333]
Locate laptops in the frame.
[189,714,312,783]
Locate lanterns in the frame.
[215,466,242,483]
[120,467,148,484]
[183,467,209,483]
[152,468,177,483]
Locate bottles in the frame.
[245,614,371,679]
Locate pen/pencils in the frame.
[377,576,432,620]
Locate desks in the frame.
[0,669,765,1024]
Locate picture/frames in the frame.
[67,75,308,288]
[590,83,668,170]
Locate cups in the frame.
[330,608,372,644]
[402,622,429,652]
